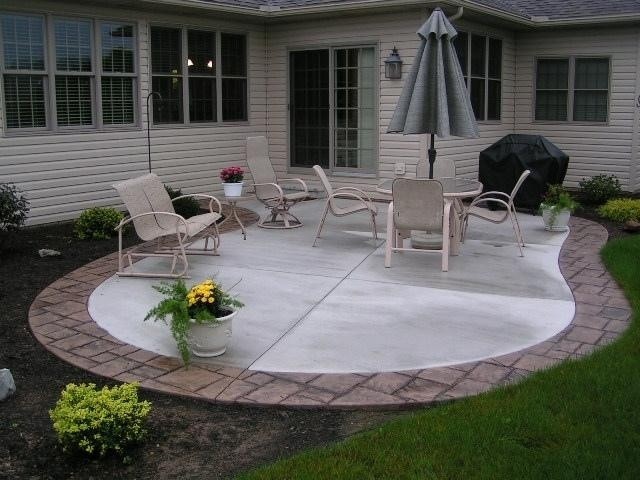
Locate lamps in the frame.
[383,46,403,79]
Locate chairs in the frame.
[112,172,222,279]
[246,136,309,229]
[312,158,531,272]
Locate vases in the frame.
[183,306,239,358]
[221,181,244,197]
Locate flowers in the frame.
[144,273,247,371]
[218,165,245,183]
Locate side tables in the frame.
[215,193,256,240]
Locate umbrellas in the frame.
[385,6,481,253]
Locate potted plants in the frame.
[537,183,580,232]
